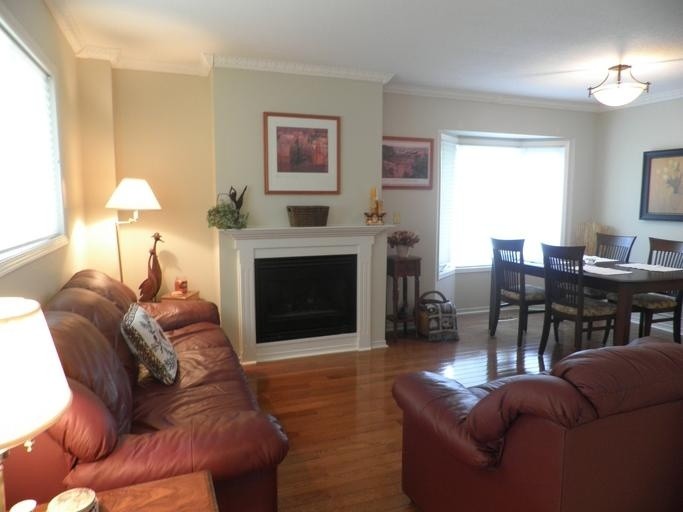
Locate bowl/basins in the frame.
[46,486,99,511]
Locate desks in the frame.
[386,256,422,334]
[11,468,219,512]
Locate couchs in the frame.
[6,268,290,512]
[389,333,683,512]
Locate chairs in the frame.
[489,235,560,349]
[558,231,637,339]
[600,237,683,347]
[538,239,621,357]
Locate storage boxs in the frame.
[286,206,331,227]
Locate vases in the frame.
[392,245,411,258]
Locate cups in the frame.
[8,498,37,511]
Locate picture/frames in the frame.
[262,111,341,194]
[638,147,683,223]
[381,134,434,191]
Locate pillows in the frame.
[118,301,183,388]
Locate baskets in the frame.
[415,290,447,338]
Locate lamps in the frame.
[588,66,651,111]
[103,176,162,232]
[0,297,74,512]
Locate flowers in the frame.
[386,231,421,249]
